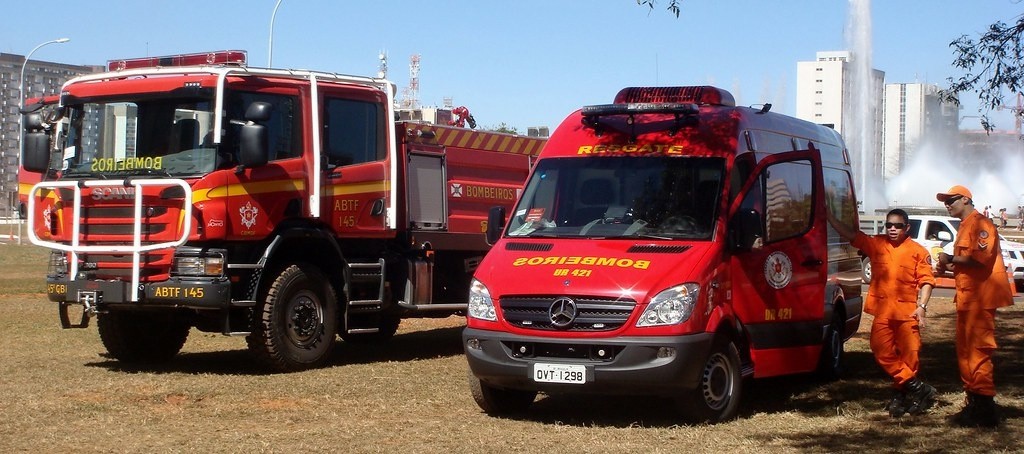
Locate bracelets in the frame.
[919,304,927,311]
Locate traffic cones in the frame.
[1005,262,1021,300]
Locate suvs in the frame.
[863,215,1024,288]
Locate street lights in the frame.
[15,37,70,246]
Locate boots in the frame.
[950,393,997,425]
[904,376,937,417]
[889,387,914,418]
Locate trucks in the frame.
[20,50,549,376]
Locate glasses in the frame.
[945,195,963,205]
[886,222,907,229]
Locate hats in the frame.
[937,185,972,199]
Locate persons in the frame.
[983,204,1024,232]
[824,205,938,415]
[935,185,1014,424]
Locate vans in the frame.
[461,85,865,425]
